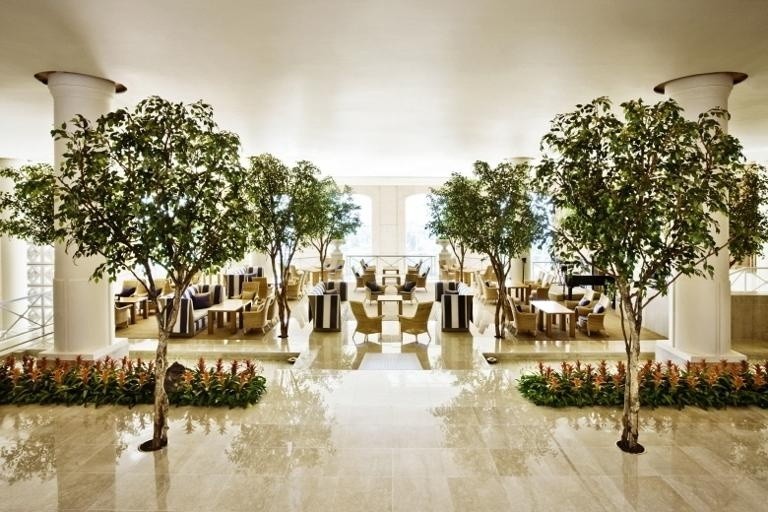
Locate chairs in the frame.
[564,288,601,325]
[397,301,434,344]
[252,295,278,326]
[506,295,538,337]
[113,253,611,299]
[115,303,133,328]
[307,294,341,333]
[440,294,473,333]
[574,293,614,338]
[241,297,271,335]
[505,302,530,326]
[350,301,385,342]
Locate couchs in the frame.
[157,283,224,339]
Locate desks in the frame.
[207,299,252,335]
[528,299,575,337]
[115,296,148,323]
[377,294,403,322]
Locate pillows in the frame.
[190,293,211,308]
[579,297,590,306]
[594,303,604,314]
